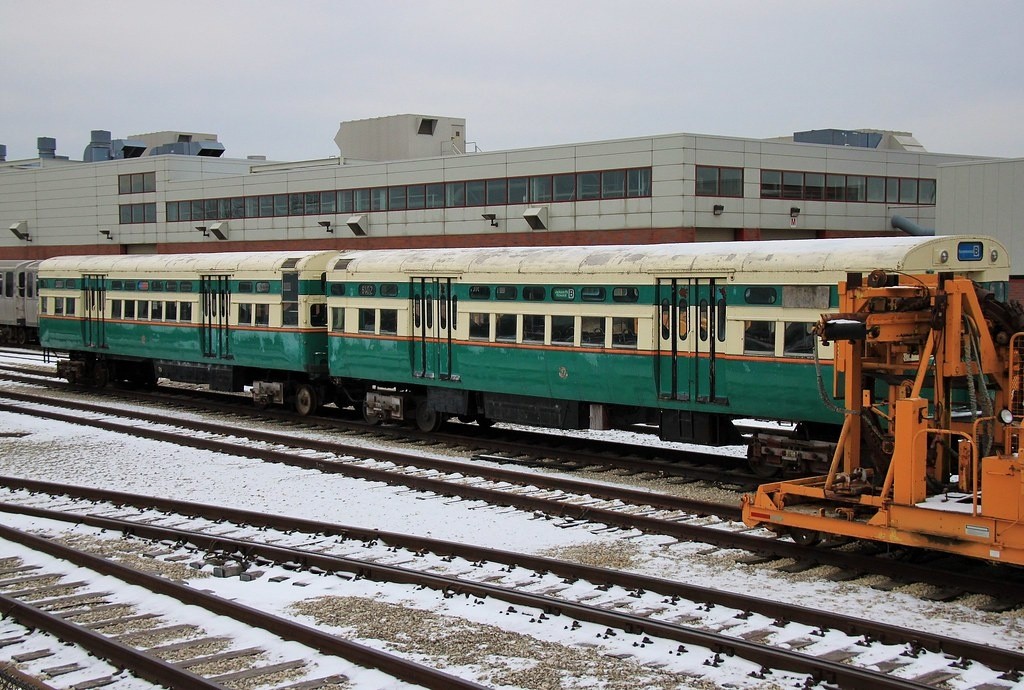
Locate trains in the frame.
[0,260,43,346]
[38,234,1010,448]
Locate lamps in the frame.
[100,230,112,241]
[22,232,32,242]
[318,221,333,233]
[790,207,800,217]
[482,214,499,227]
[714,205,724,215]
[196,227,209,237]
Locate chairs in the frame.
[536,324,638,347]
